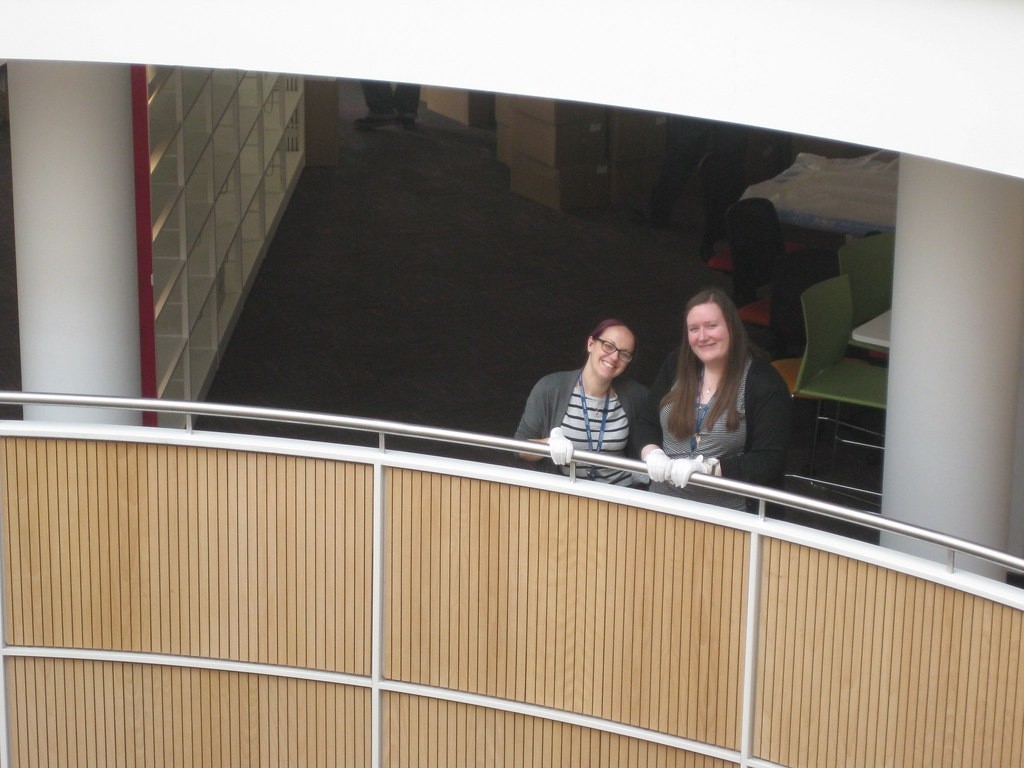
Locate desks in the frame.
[851,309,892,349]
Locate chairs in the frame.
[708,197,895,509]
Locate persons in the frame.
[708,199,804,327]
[514,318,658,490]
[627,116,749,271]
[631,290,792,521]
[354,78,420,130]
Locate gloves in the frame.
[644,449,673,484]
[548,427,574,466]
[668,455,707,489]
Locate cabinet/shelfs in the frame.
[128,63,304,432]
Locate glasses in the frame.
[596,337,634,363]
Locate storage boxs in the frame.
[493,92,668,210]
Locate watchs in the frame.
[708,458,719,475]
